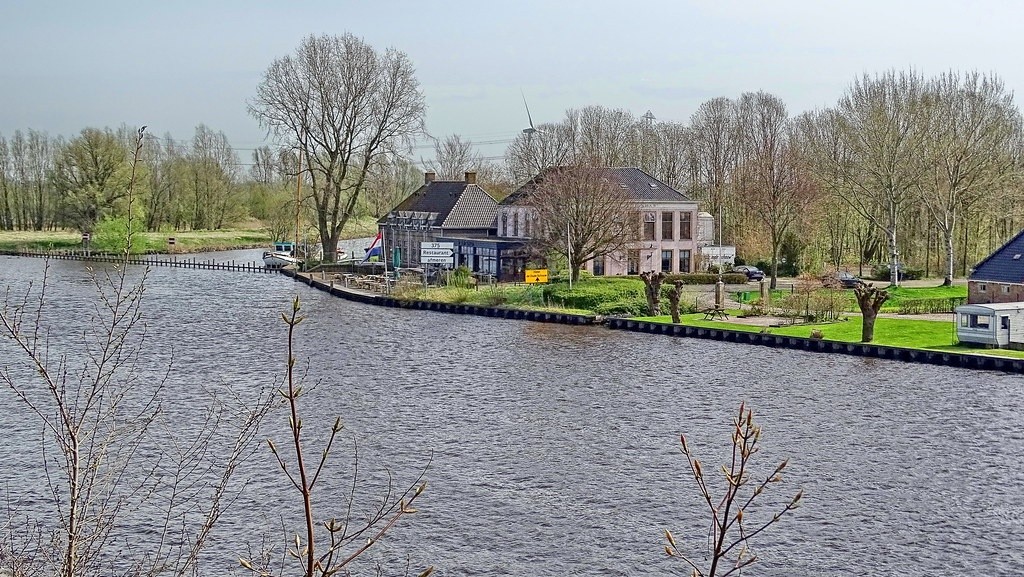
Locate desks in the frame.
[704,308,729,321]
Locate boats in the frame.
[262,240,347,268]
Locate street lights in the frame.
[690,132,722,282]
[518,266,521,287]
[523,127,571,288]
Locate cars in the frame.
[821,271,864,290]
[871,264,891,277]
[723,266,764,282]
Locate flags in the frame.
[361,230,382,263]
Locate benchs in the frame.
[719,313,729,315]
[704,313,715,316]
[336,272,399,294]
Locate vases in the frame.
[714,304,719,309]
